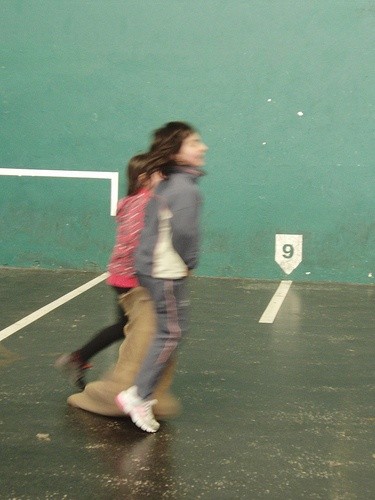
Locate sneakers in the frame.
[59,352,91,392]
[116,387,160,433]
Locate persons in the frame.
[119,119,210,432]
[54,152,164,389]
